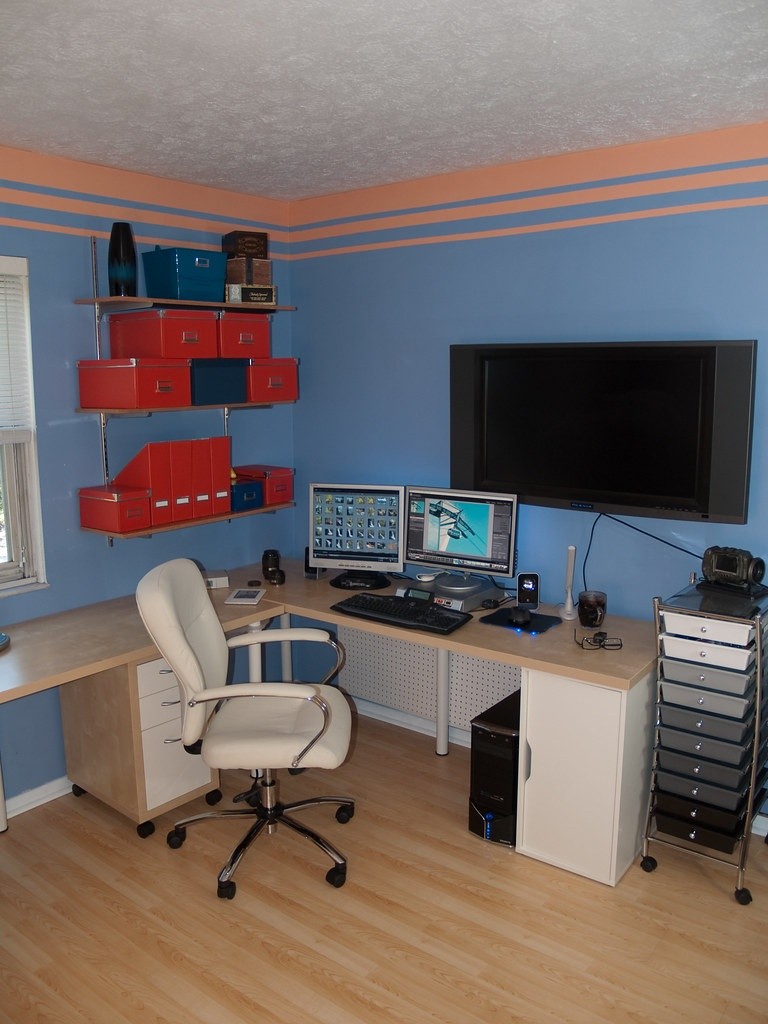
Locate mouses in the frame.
[509,606,532,625]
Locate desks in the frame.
[0,589,284,837]
[226,557,660,887]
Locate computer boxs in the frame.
[468,688,522,848]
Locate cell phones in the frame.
[516,572,540,611]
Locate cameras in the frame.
[702,545,765,588]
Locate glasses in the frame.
[573,629,622,650]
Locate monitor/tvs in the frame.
[450,339,760,525]
[308,482,519,591]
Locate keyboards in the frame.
[330,593,473,635]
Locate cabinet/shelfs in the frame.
[73,236,297,548]
[641,572,768,905]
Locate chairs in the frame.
[135,559,355,899]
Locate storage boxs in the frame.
[77,230,299,411]
[79,436,295,533]
[650,606,768,856]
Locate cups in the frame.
[578,591,607,629]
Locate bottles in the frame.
[262,549,279,579]
[108,222,138,297]
